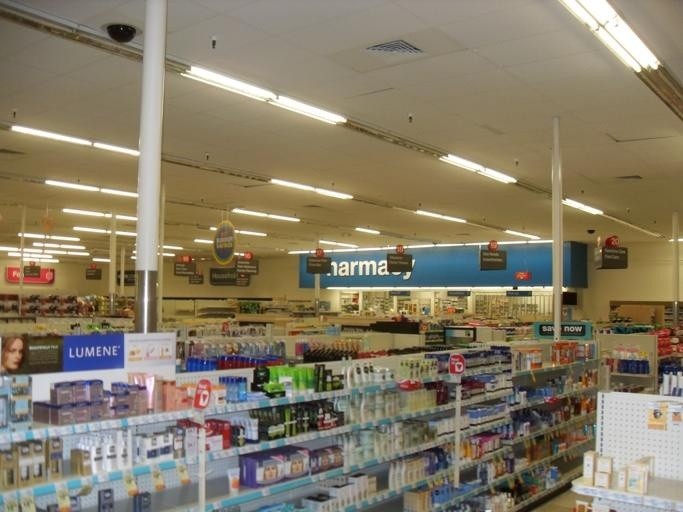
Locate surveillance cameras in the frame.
[107,25,135,44]
[587,226,596,234]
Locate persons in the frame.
[0,336,25,375]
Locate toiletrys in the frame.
[657,360,682,396]
[600,344,652,375]
[0,340,598,512]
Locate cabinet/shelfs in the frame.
[0,290,682,511]
